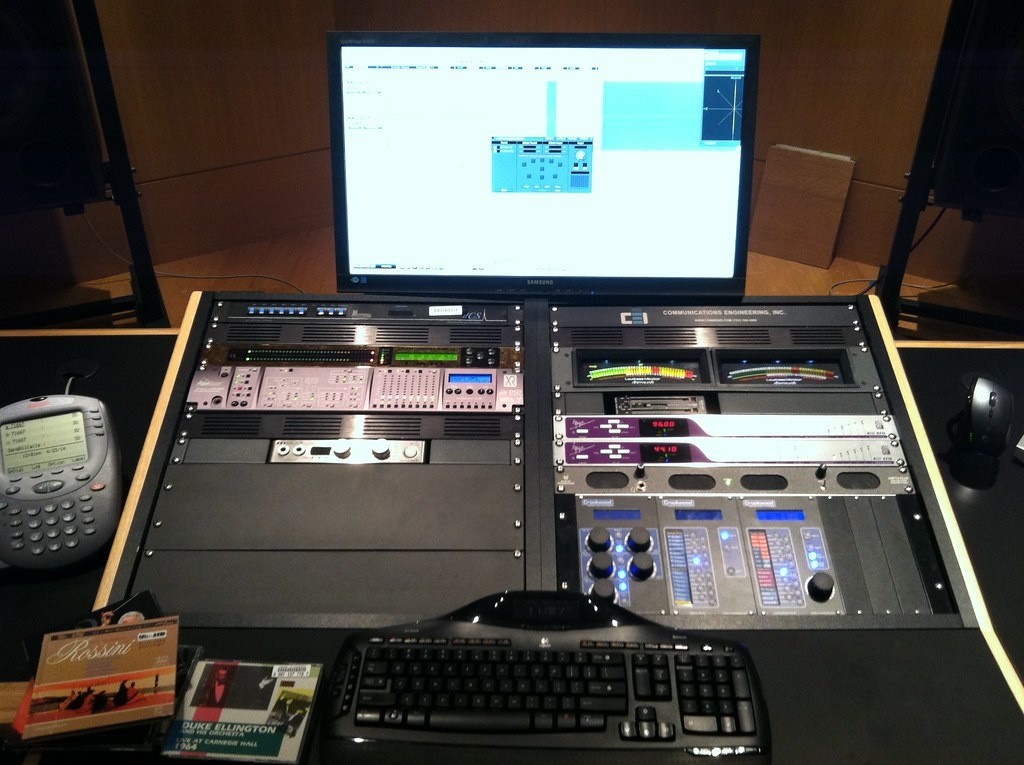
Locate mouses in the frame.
[968,377,1011,458]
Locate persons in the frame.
[59,680,139,714]
[202,669,229,706]
[100,611,145,627]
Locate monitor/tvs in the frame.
[326,31,759,302]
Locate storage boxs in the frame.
[748,147,856,272]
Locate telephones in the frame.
[0,395,122,574]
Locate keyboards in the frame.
[320,590,771,765]
